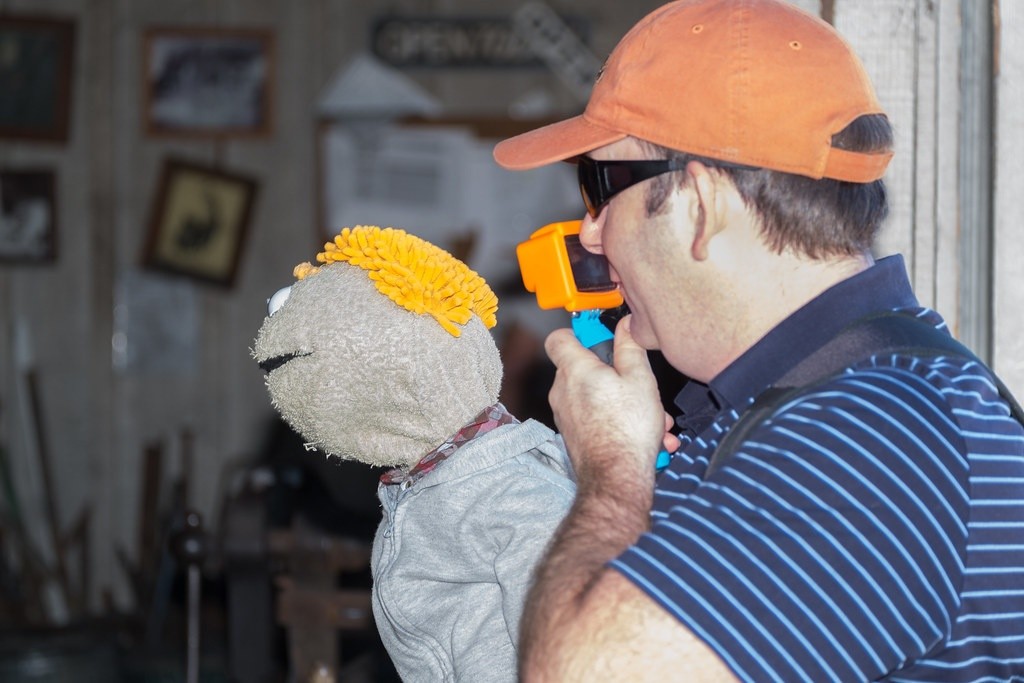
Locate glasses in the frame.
[577,151,762,225]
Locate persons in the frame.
[493,2,1024,683]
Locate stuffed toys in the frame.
[249,222,575,683]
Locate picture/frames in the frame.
[0,165,56,267]
[0,9,78,145]
[139,153,261,292]
[144,23,275,139]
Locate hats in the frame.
[492,0,895,185]
